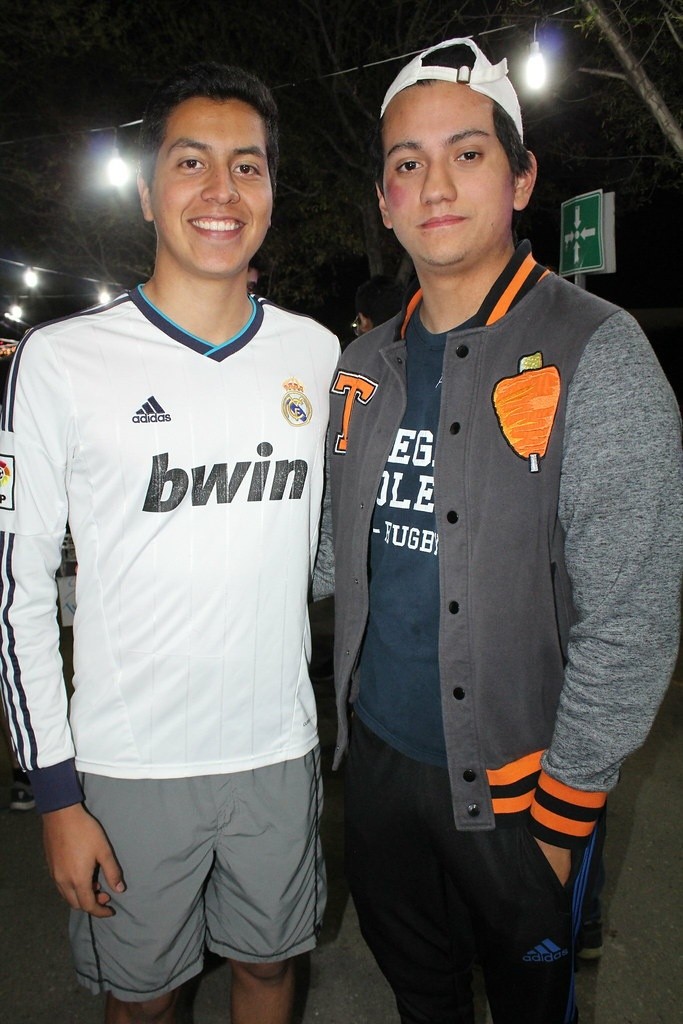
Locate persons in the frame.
[0,61,343,1024]
[305,40,683,1024]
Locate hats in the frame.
[379,38,524,136]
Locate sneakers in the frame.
[10,781,35,810]
